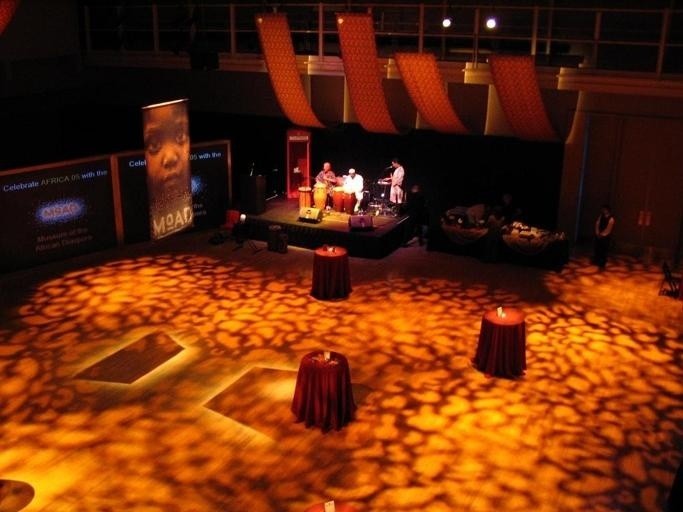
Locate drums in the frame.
[333,187,343,214]
[343,191,356,215]
[314,184,326,209]
[298,186,311,208]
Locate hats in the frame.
[349,168,355,173]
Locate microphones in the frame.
[385,166,392,170]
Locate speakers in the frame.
[298,207,322,223]
[348,215,373,231]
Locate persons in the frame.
[487,192,516,226]
[383,158,407,206]
[342,168,365,213]
[313,161,338,211]
[143,101,191,210]
[589,205,615,273]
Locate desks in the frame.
[470,307,525,378]
[291,350,357,430]
[310,246,353,301]
